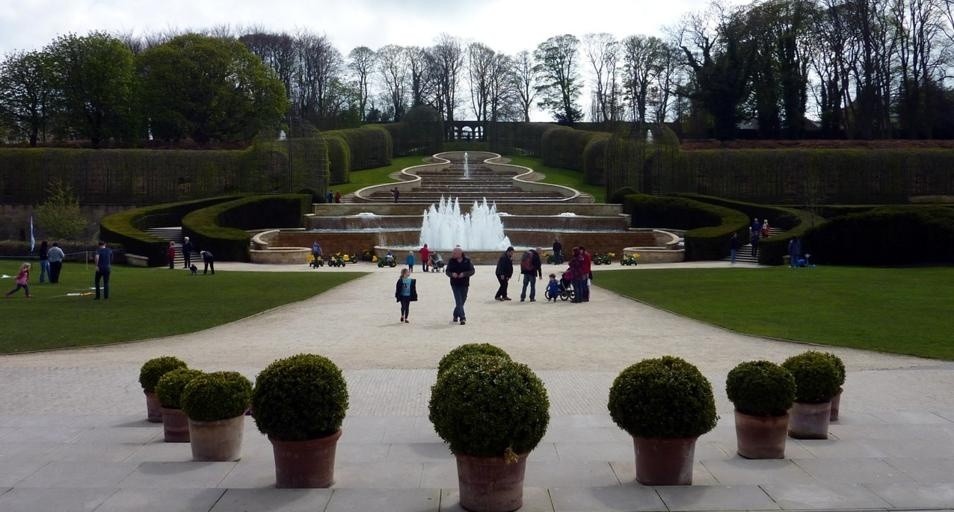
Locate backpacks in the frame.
[520,249,537,272]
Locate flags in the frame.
[44,243,65,283]
[29,217,36,254]
[92,239,113,300]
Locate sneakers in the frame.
[453,316,458,322]
[520,297,590,303]
[460,318,466,325]
[400,317,410,323]
[502,296,512,300]
[496,296,504,301]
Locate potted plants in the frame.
[140,355,250,464]
[251,355,348,490]
[607,355,718,486]
[429,355,549,512]
[725,349,849,462]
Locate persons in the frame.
[333,191,342,203]
[180,236,191,268]
[390,187,399,203]
[568,247,584,303]
[5,262,33,299]
[310,240,322,269]
[730,217,803,267]
[326,189,333,202]
[189,264,197,273]
[494,246,513,300]
[199,250,215,275]
[545,273,558,302]
[406,250,414,273]
[418,243,434,272]
[552,239,561,264]
[578,247,592,302]
[385,248,393,261]
[518,247,542,302]
[394,268,417,323]
[167,240,175,269]
[445,244,474,325]
[38,240,51,283]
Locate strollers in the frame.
[544,273,577,301]
[429,254,445,273]
